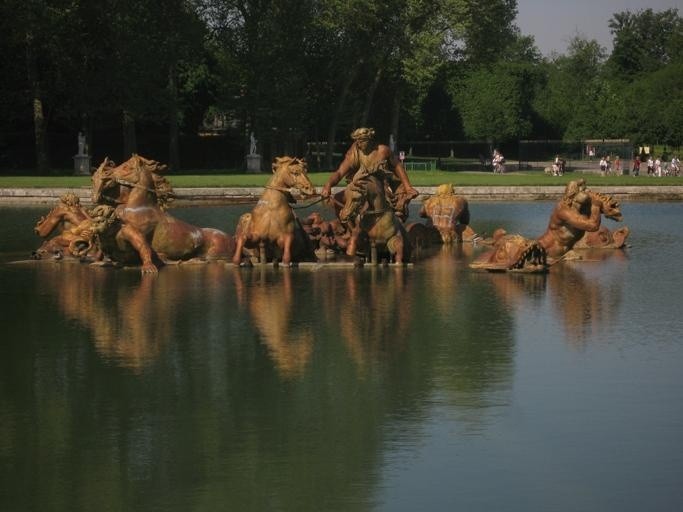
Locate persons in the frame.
[77,132,85,155]
[89,205,157,273]
[418,183,470,243]
[586,142,680,177]
[491,149,504,176]
[552,154,566,177]
[535,178,620,258]
[321,128,419,198]
[250,132,257,156]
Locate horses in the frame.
[35,154,254,275]
[339,161,410,265]
[232,156,317,266]
